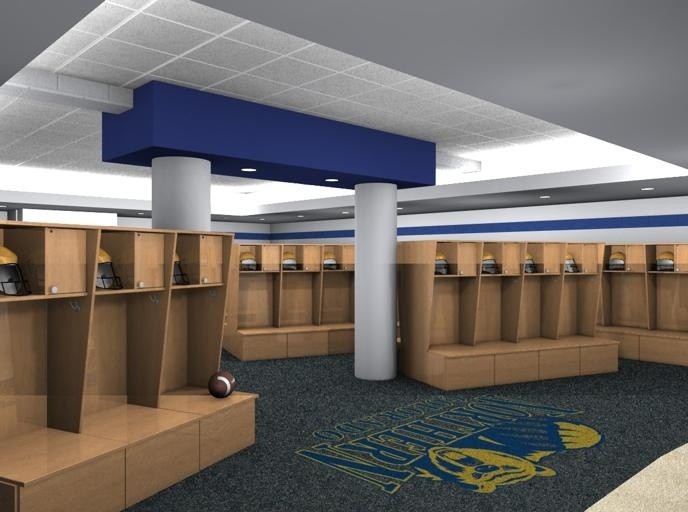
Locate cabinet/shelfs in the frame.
[598,244,688,367]
[396,240,620,391]
[224,243,353,360]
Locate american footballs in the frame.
[209,372,236,398]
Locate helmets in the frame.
[0,244,33,296]
[283,251,297,270]
[525,252,535,273]
[565,254,580,273]
[482,252,498,274]
[609,252,626,270]
[435,250,448,275]
[656,251,674,270]
[239,252,257,270]
[96,249,124,289]
[173,253,191,285]
[324,253,337,270]
